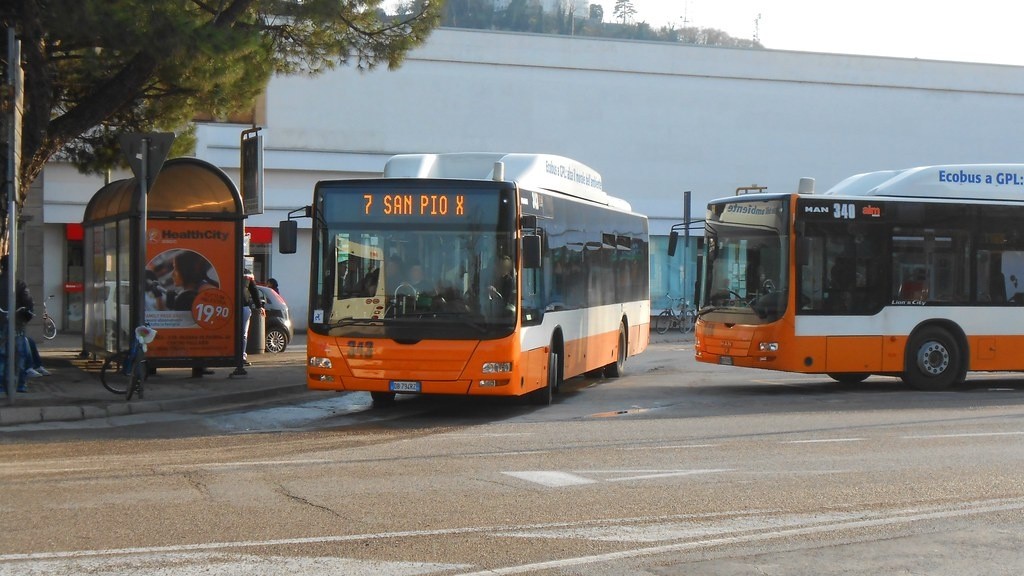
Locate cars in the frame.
[251,285,293,354]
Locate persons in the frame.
[152,251,213,311]
[759,272,767,287]
[721,279,730,298]
[473,255,514,304]
[242,276,265,364]
[267,278,279,294]
[369,254,437,296]
[902,268,927,299]
[325,260,348,297]
[0,254,52,393]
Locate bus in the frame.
[667,163,1024,391]
[278,152,652,406]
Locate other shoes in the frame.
[243,360,251,366]
[16,382,28,392]
[0,385,9,398]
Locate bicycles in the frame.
[101,320,153,400]
[35,295,57,340]
[0,306,39,395]
[656,290,696,334]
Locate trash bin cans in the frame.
[246,308,266,354]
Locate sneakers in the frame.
[26,367,42,378]
[34,366,52,376]
